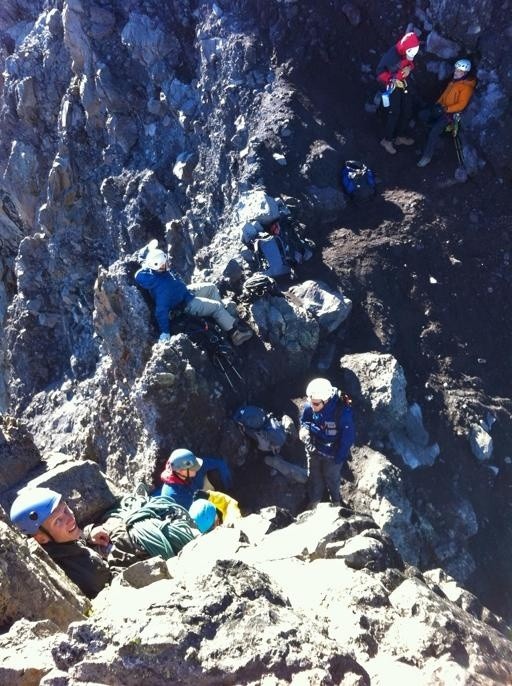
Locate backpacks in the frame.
[253,235,291,278]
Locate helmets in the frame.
[306,378,333,402]
[10,487,63,536]
[146,248,168,271]
[188,498,217,534]
[169,448,204,472]
[454,59,472,72]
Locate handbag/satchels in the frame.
[238,191,281,226]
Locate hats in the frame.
[401,32,419,61]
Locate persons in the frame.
[299,377,354,502]
[374,31,420,155]
[135,250,253,346]
[188,488,241,535]
[417,58,477,167]
[10,488,112,603]
[160,448,229,510]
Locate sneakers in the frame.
[380,136,415,155]
[230,328,254,347]
[417,156,431,167]
